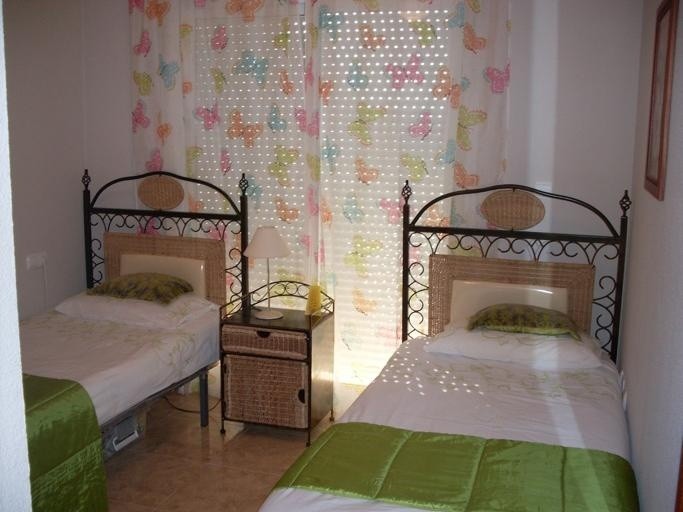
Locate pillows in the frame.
[54,288,217,332]
[88,272,195,305]
[465,303,581,341]
[422,325,605,371]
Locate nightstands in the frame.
[217,276,334,445]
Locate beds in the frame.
[17,169,251,512]
[260,179,639,512]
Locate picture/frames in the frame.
[642,0,680,203]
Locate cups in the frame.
[304,284,323,318]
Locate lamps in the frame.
[241,226,290,322]
